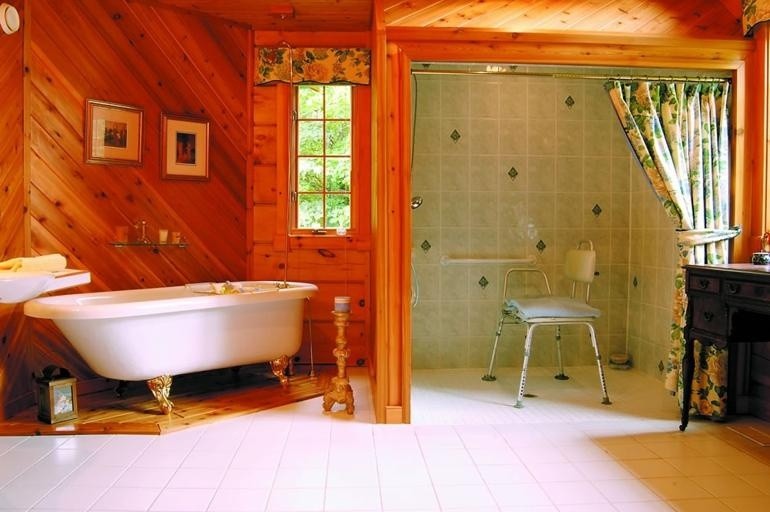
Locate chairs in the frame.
[479,239,612,408]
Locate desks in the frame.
[678,263,770,432]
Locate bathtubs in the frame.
[24,280,319,415]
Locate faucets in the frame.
[138,219,151,247]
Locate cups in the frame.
[159,230,168,246]
[116,227,127,243]
[172,233,180,243]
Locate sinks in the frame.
[0,268,90,304]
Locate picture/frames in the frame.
[159,111,211,181]
[84,98,144,168]
